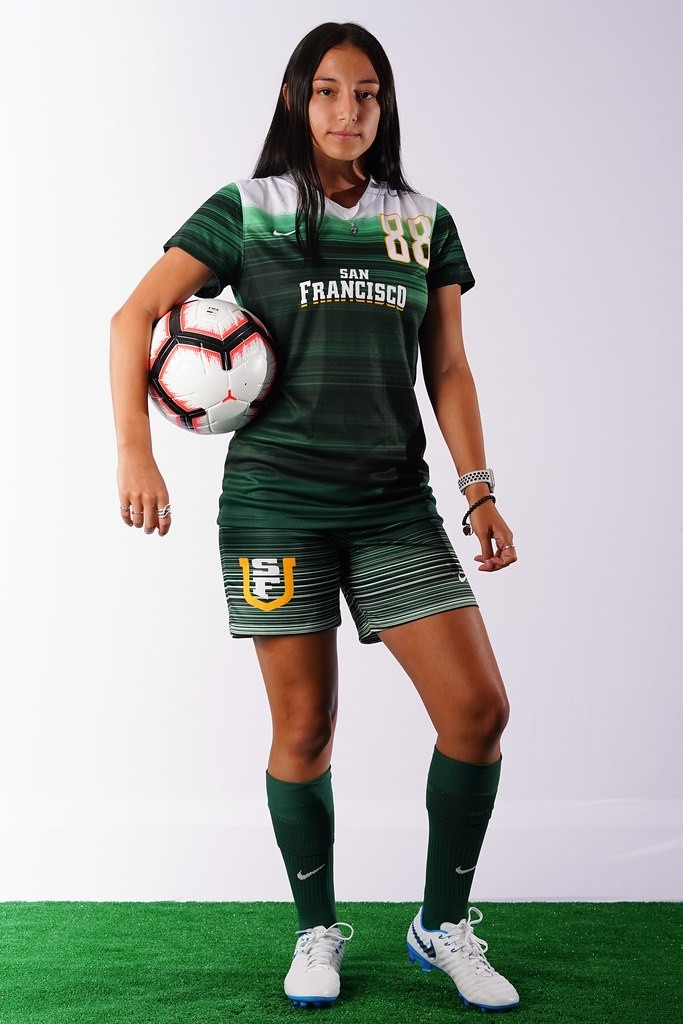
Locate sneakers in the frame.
[282,921,354,1009]
[405,903,519,1012]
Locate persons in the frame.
[109,22,520,1007]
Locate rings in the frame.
[158,504,172,519]
[132,511,143,514]
[119,507,130,512]
[502,545,515,550]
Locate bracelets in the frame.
[461,495,496,536]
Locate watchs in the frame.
[458,468,495,495]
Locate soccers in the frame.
[148,299,274,436]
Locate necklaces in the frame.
[324,195,361,233]
